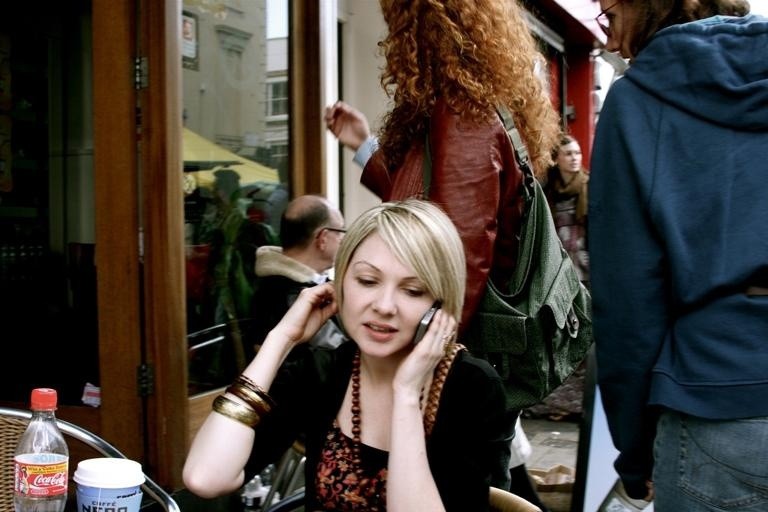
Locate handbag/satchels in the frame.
[419,79,595,414]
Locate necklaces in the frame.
[350,338,463,511]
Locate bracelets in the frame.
[213,396,261,427]
[239,374,270,401]
[228,386,276,415]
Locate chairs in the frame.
[0,411,179,512]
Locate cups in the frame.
[72,457,146,512]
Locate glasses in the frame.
[596,2,628,39]
[316,225,349,239]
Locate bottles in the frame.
[13,388,68,512]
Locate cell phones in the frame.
[412,299,441,345]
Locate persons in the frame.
[543,135,587,284]
[184,196,511,512]
[252,195,349,427]
[327,0,550,512]
[585,0,768,512]
[203,171,249,392]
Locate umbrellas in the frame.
[180,127,281,195]
